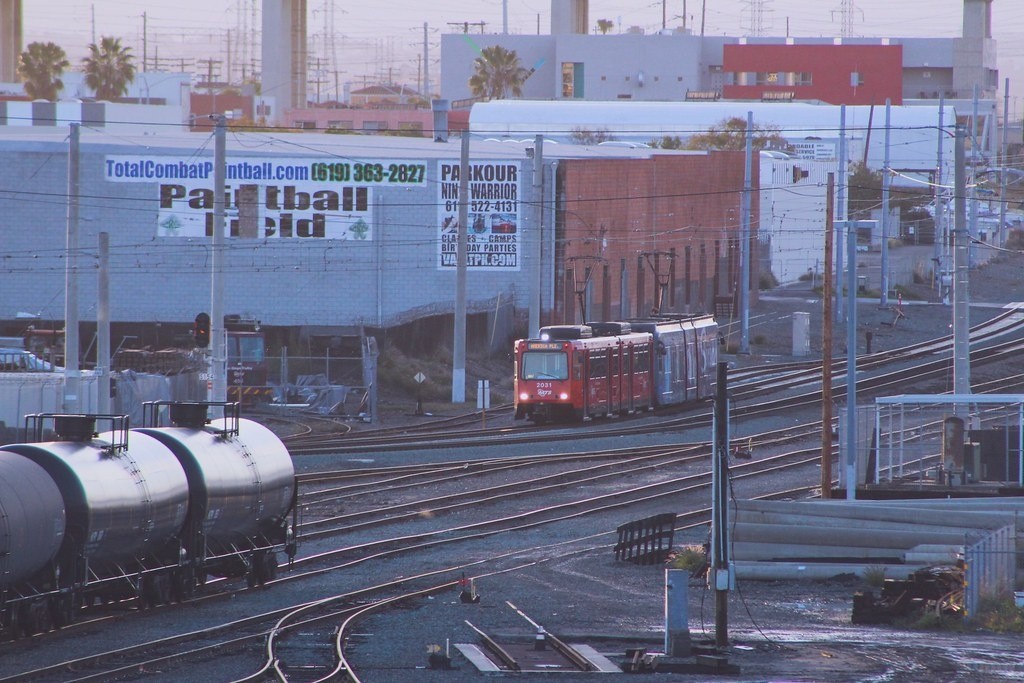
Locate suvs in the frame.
[1,348,68,374]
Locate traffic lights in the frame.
[195,312,210,348]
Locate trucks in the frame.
[0,329,67,366]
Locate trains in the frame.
[1,398,303,648]
[506,248,727,432]
